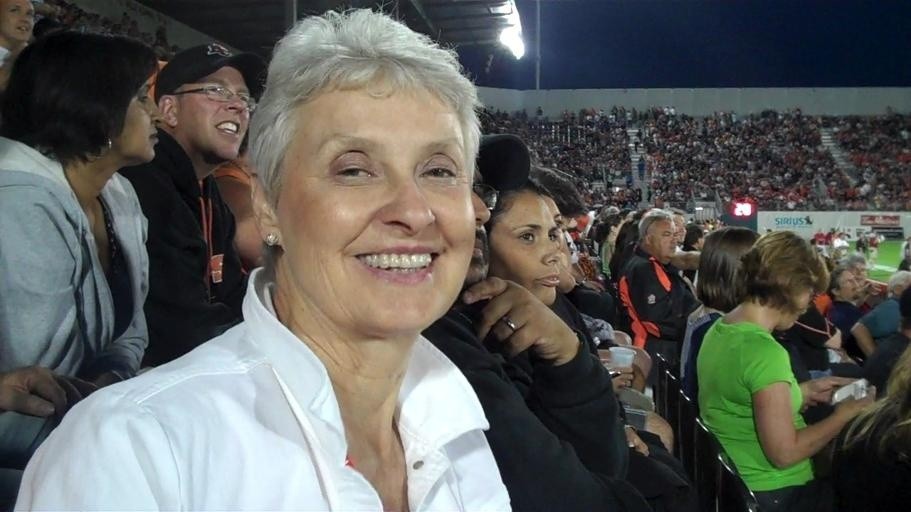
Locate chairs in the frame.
[648,347,761,511]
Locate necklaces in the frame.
[230,159,252,179]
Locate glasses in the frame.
[167,86,256,111]
[472,184,500,210]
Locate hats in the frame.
[154,43,268,109]
[476,134,530,191]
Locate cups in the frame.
[608,346,636,373]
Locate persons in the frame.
[0,25,165,512]
[14,8,514,512]
[0,365,102,512]
[213,109,268,273]
[0,0,183,91]
[116,41,256,369]
[417,102,909,512]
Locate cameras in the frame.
[831,379,869,407]
